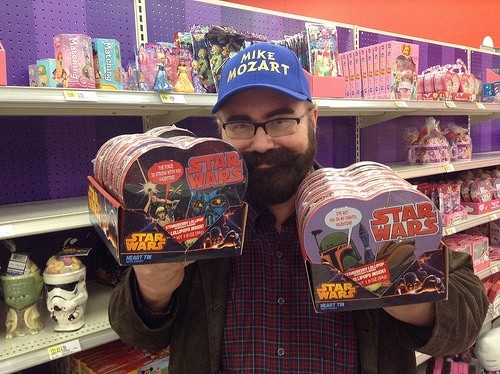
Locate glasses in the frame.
[218,107,312,139]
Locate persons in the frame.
[108,43,489,374]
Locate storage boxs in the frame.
[173,215,175,217]
[88,176,248,266]
[296,215,448,314]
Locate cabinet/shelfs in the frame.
[1,0,500,374]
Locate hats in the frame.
[211,42,312,115]
